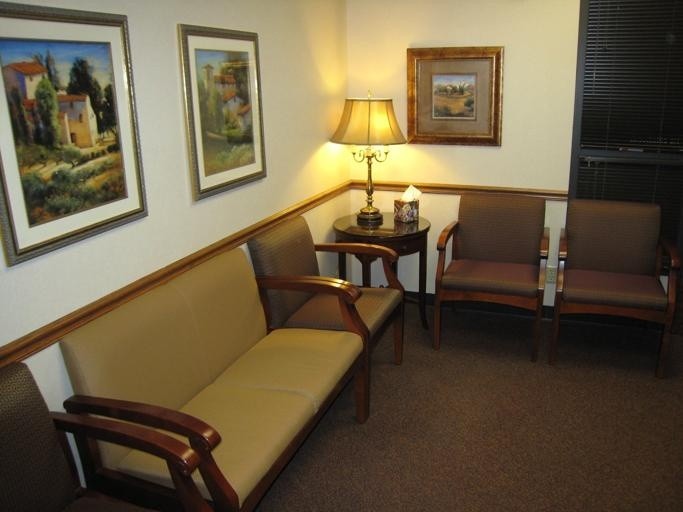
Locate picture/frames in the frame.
[173,20,271,208]
[403,43,504,152]
[0,2,151,272]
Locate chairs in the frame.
[247,214,408,421]
[0,356,221,511]
[550,194,681,380]
[431,188,551,365]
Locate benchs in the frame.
[57,244,370,511]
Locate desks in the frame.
[330,209,431,337]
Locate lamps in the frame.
[325,90,408,229]
